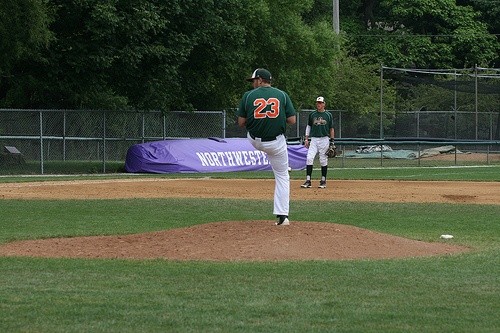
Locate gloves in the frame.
[329,138,334,146]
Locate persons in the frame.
[239,68,297,225]
[300,97,334,188]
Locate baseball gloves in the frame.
[325,138,337,158]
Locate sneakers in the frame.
[317,181,327,189]
[300,180,312,188]
[274,214,290,227]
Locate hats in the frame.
[316,96,325,102]
[246,68,272,82]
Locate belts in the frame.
[249,132,277,142]
